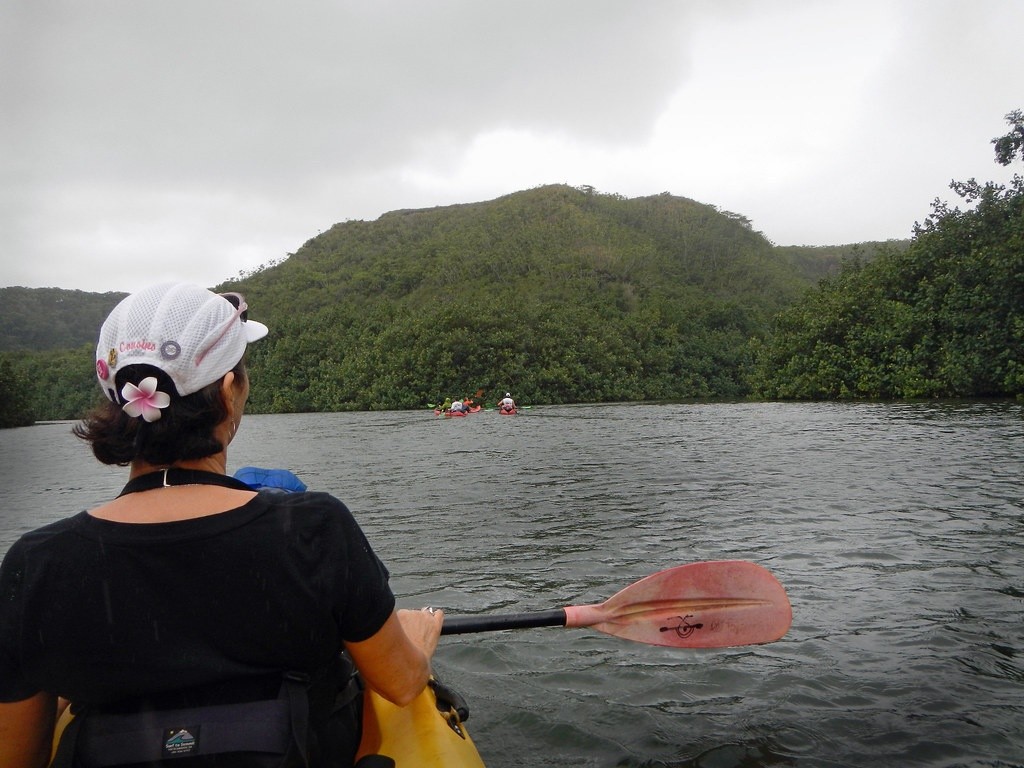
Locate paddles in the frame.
[438,561,793,648]
[427,403,438,409]
[485,406,532,412]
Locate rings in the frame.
[421,606,432,613]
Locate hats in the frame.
[95,279,269,404]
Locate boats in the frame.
[434,405,481,417]
[500,405,517,415]
[484,409,495,412]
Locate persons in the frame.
[463,396,475,410]
[439,398,451,410]
[498,393,516,411]
[0,285,444,768]
[451,396,468,412]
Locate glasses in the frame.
[195,292,249,365]
